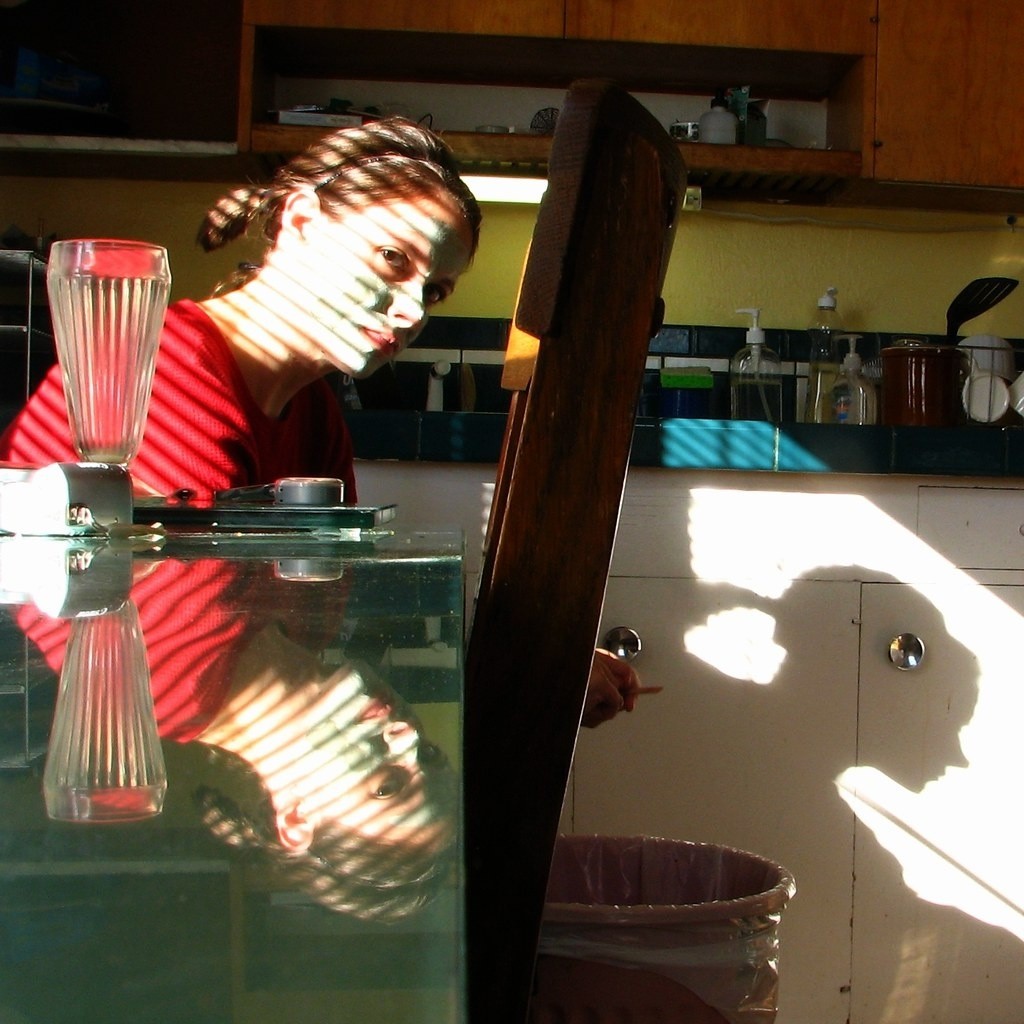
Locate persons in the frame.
[0,121,641,730]
[7,558,461,924]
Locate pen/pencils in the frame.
[621,685,663,695]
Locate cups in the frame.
[957,335,1024,423]
[663,388,709,418]
[881,346,963,427]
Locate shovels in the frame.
[945,276,1020,346]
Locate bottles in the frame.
[698,98,737,144]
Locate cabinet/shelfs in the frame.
[234,0,1024,214]
[333,408,1024,1024]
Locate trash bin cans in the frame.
[525,830,796,1024]
